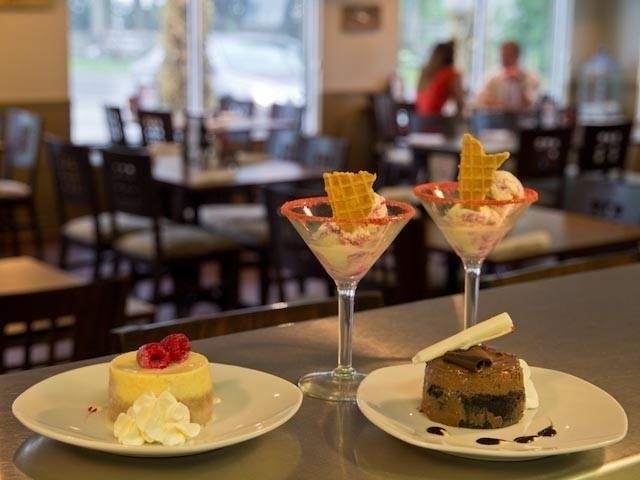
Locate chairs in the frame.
[0,89,640,353]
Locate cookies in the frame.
[457,133,510,210]
[323,170,377,232]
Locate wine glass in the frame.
[414,179,542,366]
[281,197,414,403]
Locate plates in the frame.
[11,363,304,458]
[355,362,629,462]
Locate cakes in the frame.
[109,334,212,424]
[411,312,538,429]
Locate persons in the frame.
[477,40,540,115]
[407,39,467,133]
